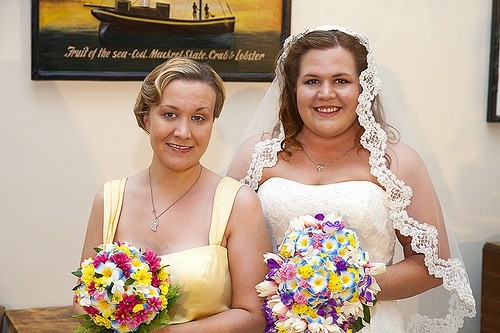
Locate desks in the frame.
[5,306,81,333]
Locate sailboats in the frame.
[84,1,235,50]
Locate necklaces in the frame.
[147,165,203,232]
[296,135,360,173]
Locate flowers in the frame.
[69,241,182,333]
[255,213,386,333]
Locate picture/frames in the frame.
[31,0,292,82]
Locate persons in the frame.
[72,58,272,333]
[226,24,475,333]
